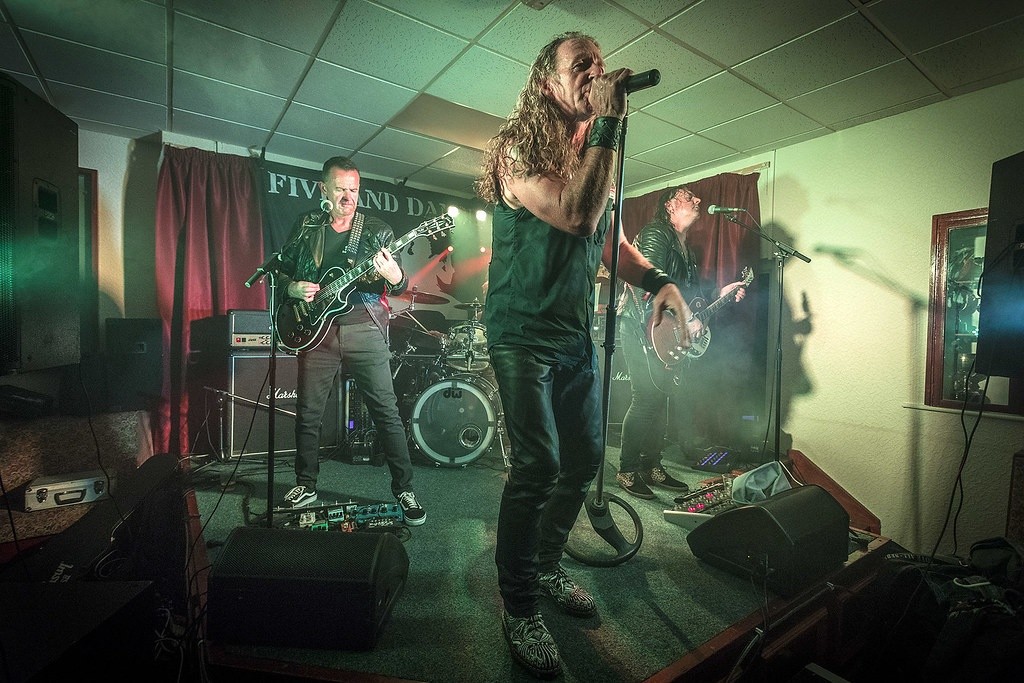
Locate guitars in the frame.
[273,213,455,353]
[646,266,755,366]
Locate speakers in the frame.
[214,351,346,460]
[206,525,410,653]
[974,149,1024,379]
[686,485,850,591]
[0,70,79,378]
[593,339,670,428]
[105,317,163,412]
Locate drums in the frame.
[409,373,504,469]
[444,321,492,372]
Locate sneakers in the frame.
[276,485,317,509]
[502,608,559,673]
[395,491,426,526]
[538,563,597,617]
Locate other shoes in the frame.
[615,470,654,499]
[639,463,689,491]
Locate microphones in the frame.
[624,68,661,95]
[707,205,746,214]
[321,200,333,213]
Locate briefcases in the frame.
[24,466,119,512]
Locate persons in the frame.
[269,157,427,527]
[614,188,746,499]
[477,31,685,675]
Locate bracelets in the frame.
[586,116,623,152]
[641,268,678,295]
[685,311,694,325]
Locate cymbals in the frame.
[389,289,450,305]
[389,324,444,354]
[453,303,486,311]
